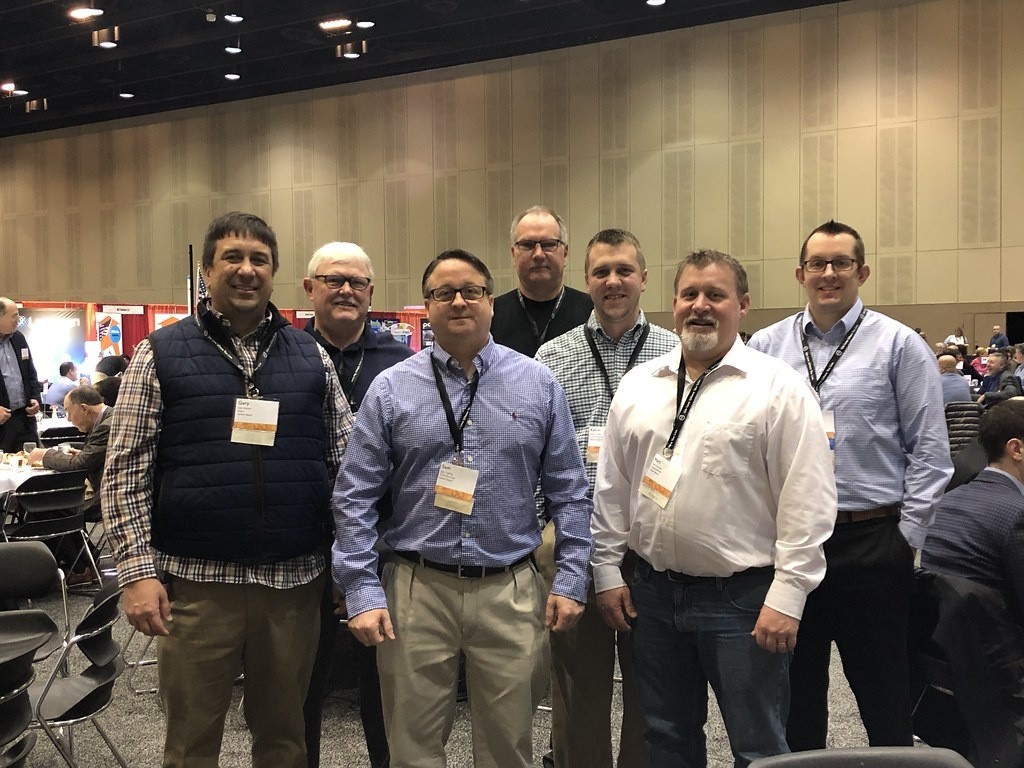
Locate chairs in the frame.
[0,422,162,768]
[945,401,983,460]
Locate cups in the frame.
[57,443,71,454]
[23,442,36,454]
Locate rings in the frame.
[778,643,786,645]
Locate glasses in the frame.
[802,257,861,272]
[315,274,373,291]
[514,239,565,252]
[426,286,488,302]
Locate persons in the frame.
[28,386,115,590]
[42,354,131,407]
[911,324,1024,629]
[532,229,683,768]
[489,204,593,359]
[743,220,955,752]
[301,241,420,768]
[330,248,594,768]
[0,296,42,453]
[99,211,356,768]
[590,250,838,768]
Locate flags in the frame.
[198,269,207,302]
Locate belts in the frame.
[835,505,899,524]
[393,548,532,580]
[634,553,767,583]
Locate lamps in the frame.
[0,0,376,116]
